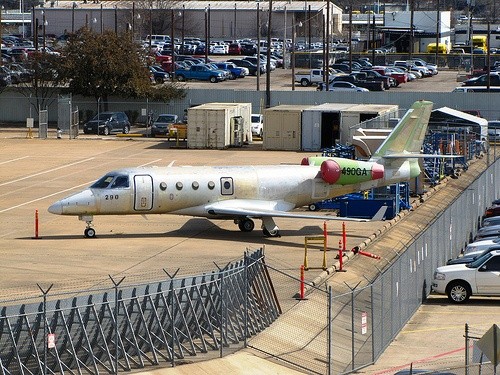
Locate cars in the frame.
[460,72,500,86]
[433,249,500,304]
[251,114,263,139]
[0,32,500,86]
[446,196,500,264]
[149,66,170,84]
[151,114,178,138]
[321,80,370,93]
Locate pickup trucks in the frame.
[175,64,225,83]
[294,68,336,87]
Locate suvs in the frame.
[83,111,131,136]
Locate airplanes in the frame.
[48,100,434,239]
